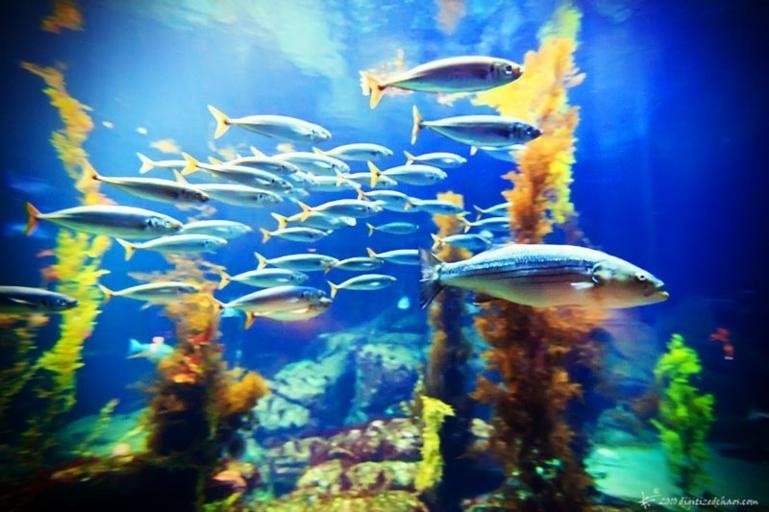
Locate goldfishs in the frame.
[357,54,529,112]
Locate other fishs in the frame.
[0,102,547,323]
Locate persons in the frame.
[696,322,735,444]
